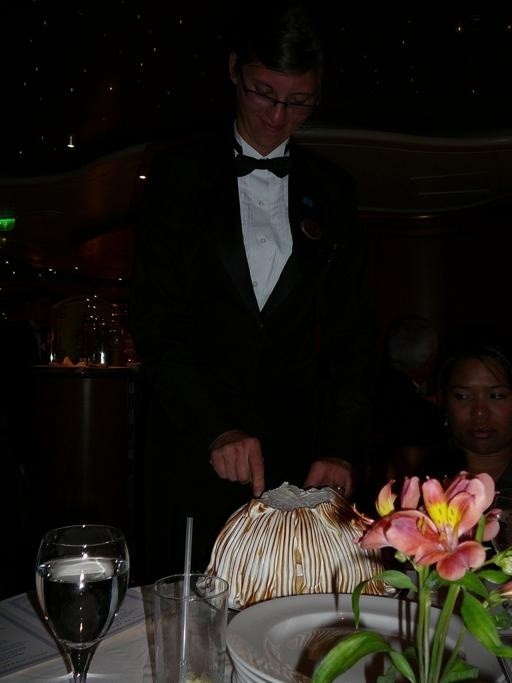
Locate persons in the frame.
[123,13,383,574]
[373,316,446,492]
[430,331,512,559]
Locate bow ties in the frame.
[233,152,295,179]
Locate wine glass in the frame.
[35,525,131,683]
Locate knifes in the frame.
[253,473,280,491]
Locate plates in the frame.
[225,593,508,683]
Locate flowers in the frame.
[310,472,512,683]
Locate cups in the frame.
[490,498,512,556]
[153,574,227,683]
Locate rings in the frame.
[332,483,345,493]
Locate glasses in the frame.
[236,60,321,113]
